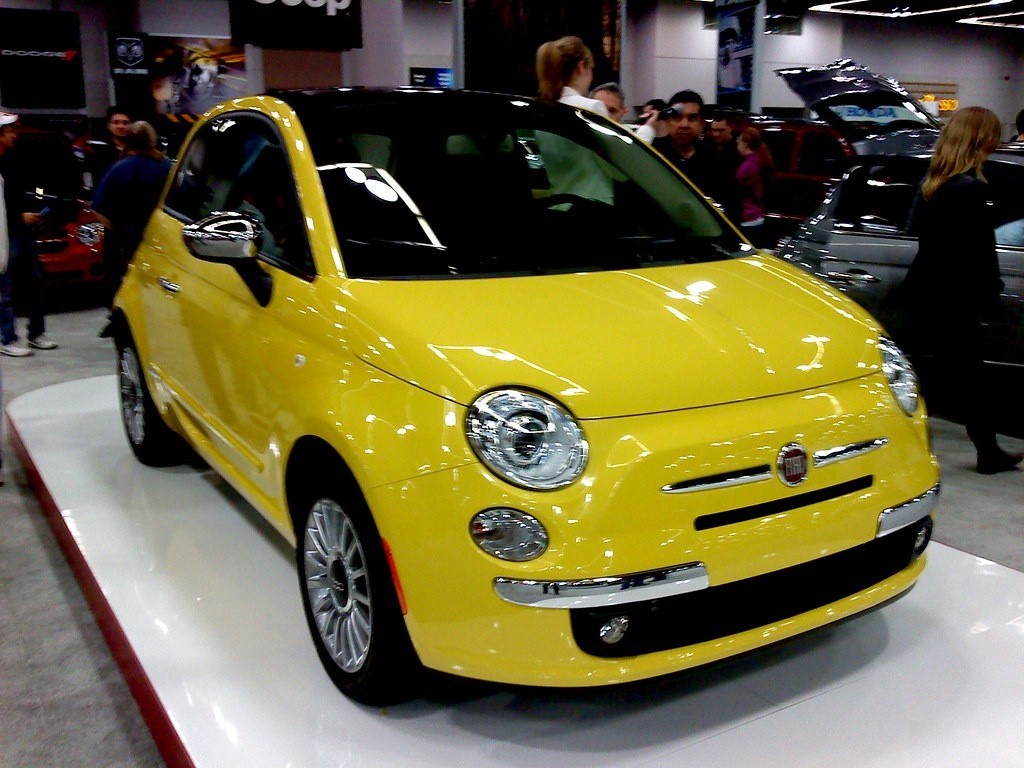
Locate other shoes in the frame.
[976,446,1024,474]
[99,323,113,339]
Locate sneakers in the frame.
[0,338,32,357]
[28,332,56,348]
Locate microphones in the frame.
[635,101,686,125]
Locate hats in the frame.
[0,111,18,131]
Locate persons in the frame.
[1011,106,1024,144]
[533,35,659,213]
[0,81,775,357]
[885,105,1024,475]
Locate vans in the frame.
[763,59,1024,431]
[695,104,849,234]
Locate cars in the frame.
[98,85,939,708]
[0,124,106,304]
